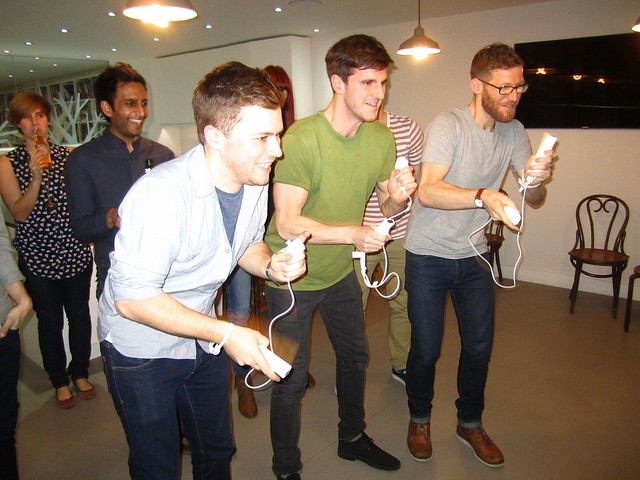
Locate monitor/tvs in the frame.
[512,32,640,131]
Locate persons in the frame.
[223,62,316,418]
[402,42,559,468]
[351,97,427,388]
[97,55,314,479]
[0,208,35,479]
[262,33,418,480]
[0,90,98,409]
[62,61,175,302]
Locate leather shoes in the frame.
[408,419,433,463]
[338,432,401,470]
[456,425,505,467]
[306,368,318,390]
[236,376,258,418]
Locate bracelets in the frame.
[208,322,235,355]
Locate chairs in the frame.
[212,276,316,419]
[485,189,509,283]
[623,265,640,333]
[568,193,630,320]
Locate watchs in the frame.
[474,187,487,209]
[265,260,286,286]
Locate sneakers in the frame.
[392,368,407,385]
[73,379,97,399]
[54,387,73,410]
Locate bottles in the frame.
[143,158,154,175]
[33,127,53,170]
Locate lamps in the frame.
[396,0,442,61]
[123,0,197,28]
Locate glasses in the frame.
[478,79,528,95]
